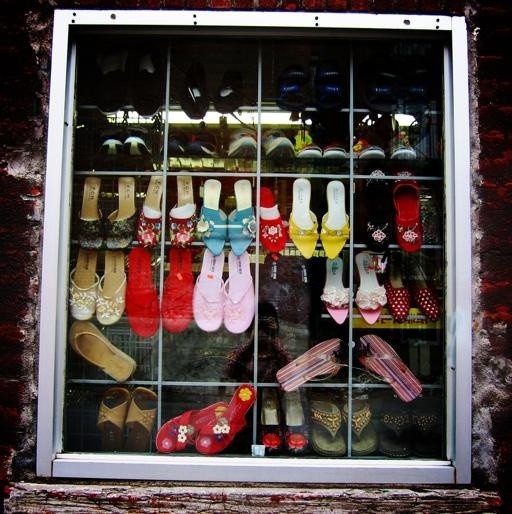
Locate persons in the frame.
[216,386,229,401]
[223,301,291,456]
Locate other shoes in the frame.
[258,388,445,459]
[98,127,418,160]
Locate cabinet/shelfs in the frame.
[36,8,470,485]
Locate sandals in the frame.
[94,35,431,115]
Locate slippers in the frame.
[78,170,442,334]
[196,385,256,455]
[70,320,136,383]
[96,386,158,454]
[154,401,228,454]
